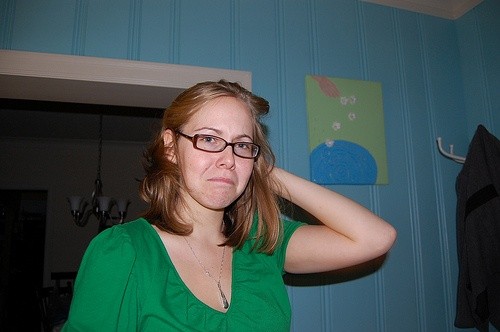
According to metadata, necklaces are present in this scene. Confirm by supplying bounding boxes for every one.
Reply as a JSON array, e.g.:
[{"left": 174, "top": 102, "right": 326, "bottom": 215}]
[{"left": 184, "top": 235, "right": 229, "bottom": 309}]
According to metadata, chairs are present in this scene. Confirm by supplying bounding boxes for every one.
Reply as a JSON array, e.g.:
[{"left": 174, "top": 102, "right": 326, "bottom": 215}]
[{"left": 44, "top": 272, "right": 78, "bottom": 331}]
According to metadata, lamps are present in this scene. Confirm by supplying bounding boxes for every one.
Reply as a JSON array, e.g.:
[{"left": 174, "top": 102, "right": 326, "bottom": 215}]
[{"left": 65, "top": 103, "right": 129, "bottom": 233}]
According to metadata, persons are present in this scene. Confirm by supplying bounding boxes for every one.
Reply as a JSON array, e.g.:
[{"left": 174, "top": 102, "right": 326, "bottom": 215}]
[{"left": 60, "top": 79, "right": 396, "bottom": 332}]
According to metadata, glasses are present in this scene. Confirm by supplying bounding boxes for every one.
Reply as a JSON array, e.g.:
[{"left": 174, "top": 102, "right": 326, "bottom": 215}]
[{"left": 175, "top": 130, "right": 262, "bottom": 159}]
